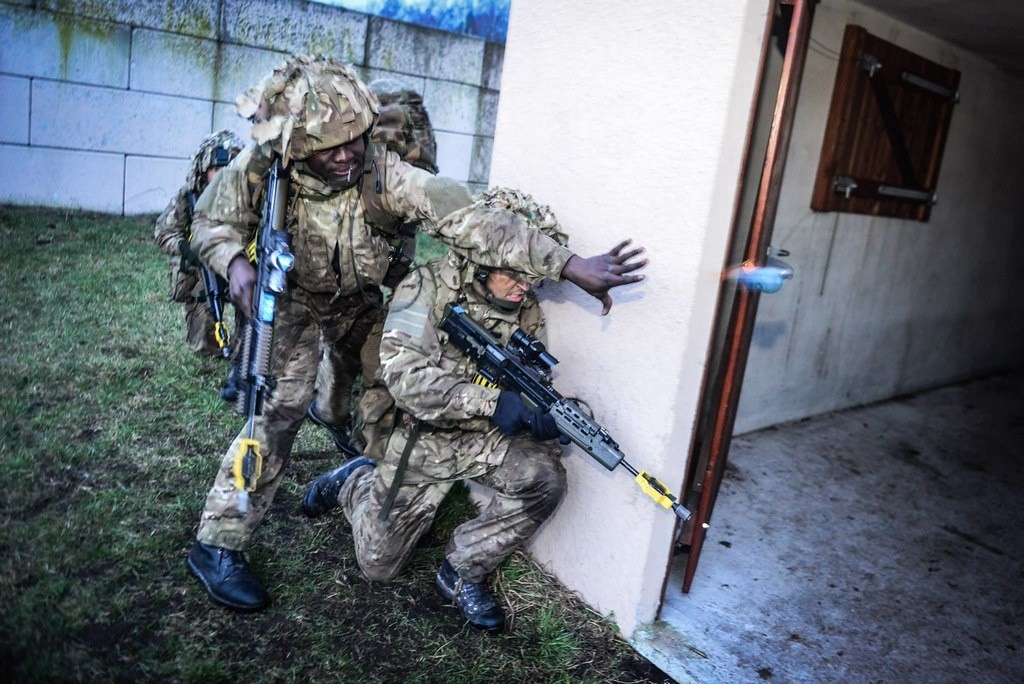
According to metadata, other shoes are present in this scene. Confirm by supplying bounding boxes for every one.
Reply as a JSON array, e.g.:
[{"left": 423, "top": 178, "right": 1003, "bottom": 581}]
[
  {"left": 436, "top": 559, "right": 510, "bottom": 635},
  {"left": 220, "top": 364, "right": 240, "bottom": 401},
  {"left": 303, "top": 457, "right": 376, "bottom": 518}
]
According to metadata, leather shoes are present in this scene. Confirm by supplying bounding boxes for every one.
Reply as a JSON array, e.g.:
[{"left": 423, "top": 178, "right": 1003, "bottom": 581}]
[
  {"left": 308, "top": 399, "right": 366, "bottom": 458},
  {"left": 187, "top": 540, "right": 271, "bottom": 612}
]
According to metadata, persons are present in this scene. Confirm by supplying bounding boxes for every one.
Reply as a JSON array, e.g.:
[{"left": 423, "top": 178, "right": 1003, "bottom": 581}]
[
  {"left": 302, "top": 189, "right": 588, "bottom": 628},
  {"left": 153, "top": 56, "right": 648, "bottom": 632}
]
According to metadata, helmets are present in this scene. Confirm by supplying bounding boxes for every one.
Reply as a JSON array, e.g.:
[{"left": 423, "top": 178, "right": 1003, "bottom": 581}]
[
  {"left": 369, "top": 78, "right": 422, "bottom": 104},
  {"left": 269, "top": 75, "right": 374, "bottom": 160},
  {"left": 468, "top": 193, "right": 562, "bottom": 299},
  {"left": 201, "top": 147, "right": 241, "bottom": 167}
]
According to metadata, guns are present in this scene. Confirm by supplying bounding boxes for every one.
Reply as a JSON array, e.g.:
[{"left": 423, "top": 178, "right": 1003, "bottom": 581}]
[
  {"left": 186, "top": 190, "right": 231, "bottom": 360},
  {"left": 230, "top": 160, "right": 291, "bottom": 495},
  {"left": 433, "top": 301, "right": 693, "bottom": 527}
]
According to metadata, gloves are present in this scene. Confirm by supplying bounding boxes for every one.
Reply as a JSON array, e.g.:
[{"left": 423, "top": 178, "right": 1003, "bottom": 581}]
[{"left": 179, "top": 242, "right": 204, "bottom": 276}]
